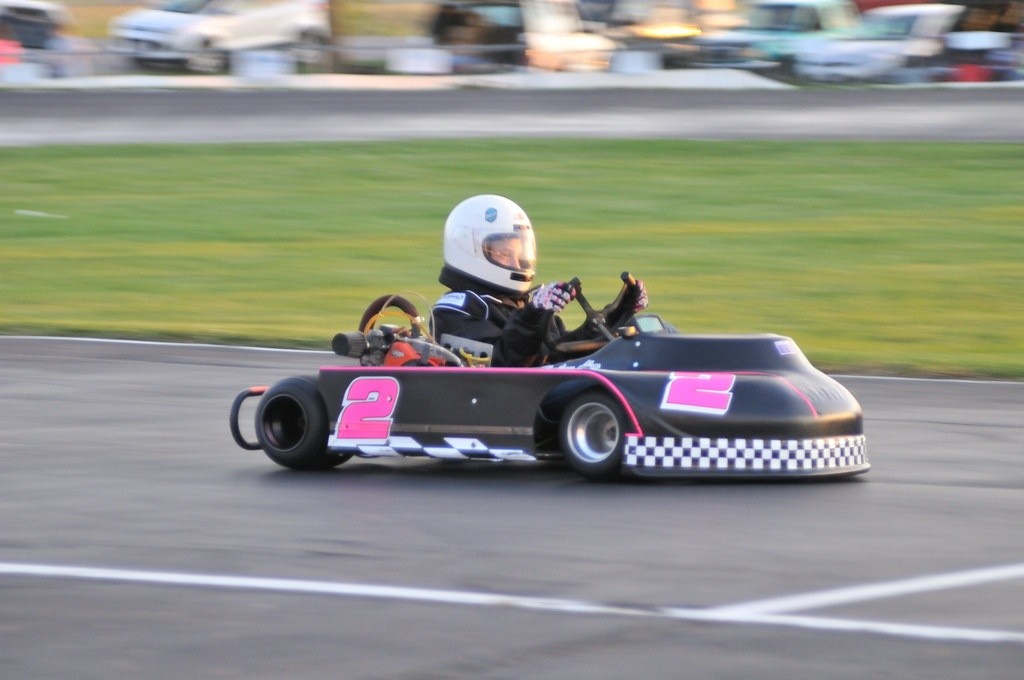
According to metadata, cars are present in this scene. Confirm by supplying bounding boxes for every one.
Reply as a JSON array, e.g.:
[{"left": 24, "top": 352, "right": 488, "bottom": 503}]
[
  {"left": 0, "top": 3, "right": 97, "bottom": 89},
  {"left": 433, "top": 0, "right": 1024, "bottom": 85},
  {"left": 103, "top": 0, "right": 333, "bottom": 76}
]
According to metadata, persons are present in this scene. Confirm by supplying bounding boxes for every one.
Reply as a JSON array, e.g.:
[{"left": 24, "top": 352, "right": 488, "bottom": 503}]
[
  {"left": 0, "top": 20, "right": 72, "bottom": 79},
  {"left": 432, "top": 0, "right": 485, "bottom": 70},
  {"left": 429, "top": 194, "right": 649, "bottom": 369}
]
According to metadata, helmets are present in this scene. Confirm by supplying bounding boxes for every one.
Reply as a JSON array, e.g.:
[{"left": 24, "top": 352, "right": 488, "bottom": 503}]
[{"left": 438, "top": 194, "right": 536, "bottom": 297}]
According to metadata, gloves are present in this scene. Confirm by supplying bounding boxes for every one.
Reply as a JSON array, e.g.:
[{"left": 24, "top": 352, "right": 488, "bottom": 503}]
[
  {"left": 527, "top": 281, "right": 576, "bottom": 313},
  {"left": 612, "top": 280, "right": 648, "bottom": 318}
]
